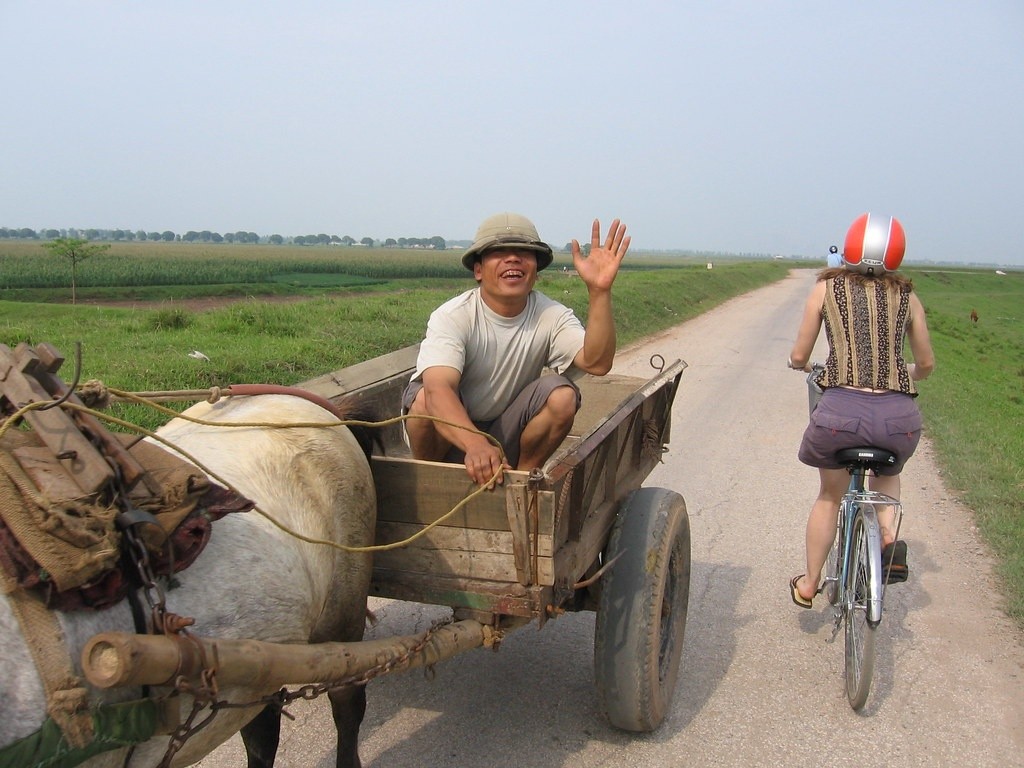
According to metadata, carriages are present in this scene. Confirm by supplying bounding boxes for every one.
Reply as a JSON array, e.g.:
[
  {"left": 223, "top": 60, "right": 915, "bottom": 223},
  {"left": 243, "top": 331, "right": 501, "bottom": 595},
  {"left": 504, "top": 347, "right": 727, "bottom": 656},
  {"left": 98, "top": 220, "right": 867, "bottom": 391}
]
[{"left": 0, "top": 334, "right": 693, "bottom": 768}]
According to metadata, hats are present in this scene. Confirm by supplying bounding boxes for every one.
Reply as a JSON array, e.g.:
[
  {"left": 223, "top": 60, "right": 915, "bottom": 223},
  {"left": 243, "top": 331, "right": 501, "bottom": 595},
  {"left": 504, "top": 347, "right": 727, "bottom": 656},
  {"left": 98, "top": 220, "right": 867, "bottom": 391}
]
[
  {"left": 829, "top": 246, "right": 837, "bottom": 253},
  {"left": 462, "top": 213, "right": 553, "bottom": 273}
]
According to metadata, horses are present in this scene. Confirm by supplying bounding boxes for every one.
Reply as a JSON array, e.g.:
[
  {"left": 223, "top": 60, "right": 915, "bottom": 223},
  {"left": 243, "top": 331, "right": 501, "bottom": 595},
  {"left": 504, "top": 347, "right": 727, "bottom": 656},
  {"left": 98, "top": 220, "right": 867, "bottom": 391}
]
[{"left": 2, "top": 389, "right": 377, "bottom": 767}]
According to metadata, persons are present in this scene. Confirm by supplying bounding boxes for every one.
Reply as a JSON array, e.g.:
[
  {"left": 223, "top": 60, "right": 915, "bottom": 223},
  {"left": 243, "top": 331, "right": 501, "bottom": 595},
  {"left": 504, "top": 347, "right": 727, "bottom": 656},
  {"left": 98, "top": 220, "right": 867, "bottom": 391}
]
[
  {"left": 789, "top": 212, "right": 935, "bottom": 609},
  {"left": 400, "top": 212, "right": 631, "bottom": 492}
]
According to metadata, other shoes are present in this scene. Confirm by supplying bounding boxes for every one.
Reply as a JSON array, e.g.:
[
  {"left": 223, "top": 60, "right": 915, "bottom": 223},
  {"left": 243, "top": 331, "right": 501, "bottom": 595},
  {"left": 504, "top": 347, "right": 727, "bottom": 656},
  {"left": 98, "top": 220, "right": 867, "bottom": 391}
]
[{"left": 881, "top": 540, "right": 908, "bottom": 584}]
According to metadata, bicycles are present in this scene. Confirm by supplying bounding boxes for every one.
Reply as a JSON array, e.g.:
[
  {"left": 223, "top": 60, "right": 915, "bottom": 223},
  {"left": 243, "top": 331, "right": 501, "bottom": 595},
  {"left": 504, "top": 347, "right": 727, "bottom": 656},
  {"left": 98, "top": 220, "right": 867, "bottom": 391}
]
[{"left": 792, "top": 361, "right": 903, "bottom": 711}]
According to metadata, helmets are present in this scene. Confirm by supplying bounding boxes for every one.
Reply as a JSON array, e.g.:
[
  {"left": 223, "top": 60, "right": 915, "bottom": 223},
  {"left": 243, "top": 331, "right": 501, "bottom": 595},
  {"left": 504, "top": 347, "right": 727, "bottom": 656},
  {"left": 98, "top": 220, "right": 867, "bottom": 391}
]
[{"left": 840, "top": 212, "right": 906, "bottom": 276}]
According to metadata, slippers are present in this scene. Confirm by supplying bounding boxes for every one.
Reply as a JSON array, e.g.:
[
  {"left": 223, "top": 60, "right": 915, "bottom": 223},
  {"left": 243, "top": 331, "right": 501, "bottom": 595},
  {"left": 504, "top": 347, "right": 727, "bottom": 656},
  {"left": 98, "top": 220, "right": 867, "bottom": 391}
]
[{"left": 789, "top": 574, "right": 812, "bottom": 609}]
